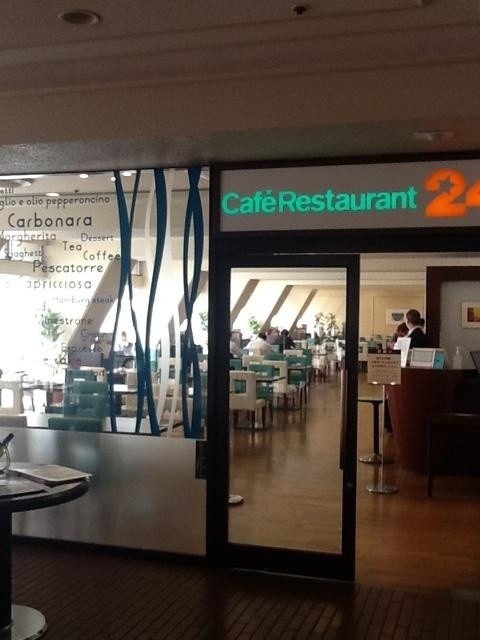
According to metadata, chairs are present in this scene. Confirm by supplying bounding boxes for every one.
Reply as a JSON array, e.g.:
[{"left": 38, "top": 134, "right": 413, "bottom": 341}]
[{"left": 0, "top": 333, "right": 393, "bottom": 432}]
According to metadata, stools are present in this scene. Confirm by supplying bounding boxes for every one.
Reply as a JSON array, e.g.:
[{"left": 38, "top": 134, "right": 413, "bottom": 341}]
[{"left": 357, "top": 399, "right": 394, "bottom": 464}]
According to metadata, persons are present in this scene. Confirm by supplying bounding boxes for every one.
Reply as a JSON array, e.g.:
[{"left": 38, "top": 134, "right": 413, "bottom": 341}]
[
  {"left": 244, "top": 330, "right": 274, "bottom": 356},
  {"left": 271, "top": 328, "right": 296, "bottom": 352},
  {"left": 230, "top": 331, "right": 243, "bottom": 359},
  {"left": 118, "top": 329, "right": 133, "bottom": 368},
  {"left": 392, "top": 322, "right": 409, "bottom": 343},
  {"left": 417, "top": 317, "right": 425, "bottom": 331},
  {"left": 385, "top": 308, "right": 425, "bottom": 355},
  {"left": 265, "top": 328, "right": 278, "bottom": 345}
]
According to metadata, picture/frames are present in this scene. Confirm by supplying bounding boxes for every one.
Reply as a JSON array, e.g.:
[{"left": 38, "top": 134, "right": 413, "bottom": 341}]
[
  {"left": 386, "top": 309, "right": 409, "bottom": 326},
  {"left": 461, "top": 302, "right": 480, "bottom": 328}
]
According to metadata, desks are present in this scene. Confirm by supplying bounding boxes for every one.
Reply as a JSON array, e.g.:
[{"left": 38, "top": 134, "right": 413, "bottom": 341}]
[{"left": 0, "top": 461, "right": 88, "bottom": 640}]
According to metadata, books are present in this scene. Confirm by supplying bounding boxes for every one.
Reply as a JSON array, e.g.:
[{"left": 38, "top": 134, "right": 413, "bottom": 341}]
[
  {"left": 5, "top": 462, "right": 95, "bottom": 486},
  {"left": 0, "top": 480, "right": 45, "bottom": 499}
]
